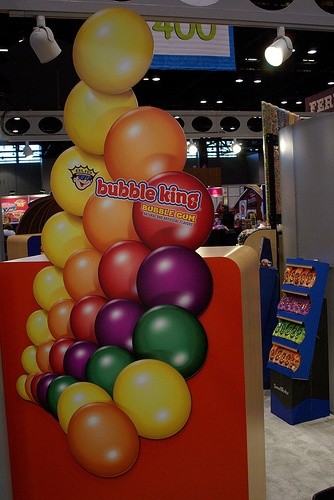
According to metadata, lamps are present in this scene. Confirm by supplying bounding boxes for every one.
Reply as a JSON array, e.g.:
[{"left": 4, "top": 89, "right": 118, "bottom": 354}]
[
  {"left": 188, "top": 139, "right": 197, "bottom": 155},
  {"left": 265, "top": 26, "right": 293, "bottom": 66},
  {"left": 23, "top": 141, "right": 33, "bottom": 157},
  {"left": 30, "top": 16, "right": 61, "bottom": 64},
  {"left": 232, "top": 138, "right": 241, "bottom": 154}
]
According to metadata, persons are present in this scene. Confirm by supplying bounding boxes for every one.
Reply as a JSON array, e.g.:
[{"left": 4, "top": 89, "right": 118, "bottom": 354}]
[
  {"left": 220, "top": 205, "right": 235, "bottom": 230},
  {"left": 3, "top": 223, "right": 15, "bottom": 236}
]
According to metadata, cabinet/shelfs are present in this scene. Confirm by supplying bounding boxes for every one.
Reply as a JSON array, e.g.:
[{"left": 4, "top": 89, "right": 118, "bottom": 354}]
[{"left": 267, "top": 257, "right": 329, "bottom": 381}]
